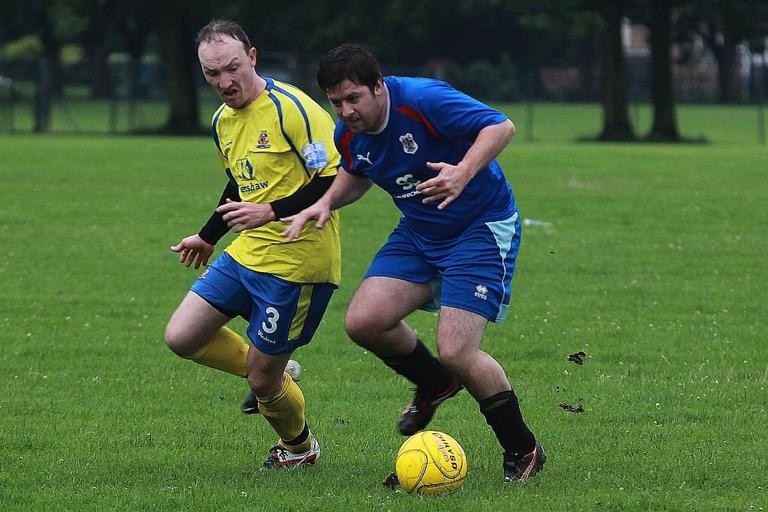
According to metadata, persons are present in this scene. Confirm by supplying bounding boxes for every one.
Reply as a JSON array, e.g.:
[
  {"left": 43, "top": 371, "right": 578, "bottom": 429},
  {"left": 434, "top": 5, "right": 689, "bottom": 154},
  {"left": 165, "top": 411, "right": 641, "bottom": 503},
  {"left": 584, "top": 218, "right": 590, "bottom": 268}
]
[
  {"left": 165, "top": 19, "right": 341, "bottom": 467},
  {"left": 279, "top": 45, "right": 545, "bottom": 483}
]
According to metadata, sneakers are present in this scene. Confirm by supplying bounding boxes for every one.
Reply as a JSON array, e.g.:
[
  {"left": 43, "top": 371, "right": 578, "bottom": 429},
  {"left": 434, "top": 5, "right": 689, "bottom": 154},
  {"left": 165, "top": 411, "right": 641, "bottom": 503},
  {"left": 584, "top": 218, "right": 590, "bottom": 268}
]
[
  {"left": 502, "top": 441, "right": 546, "bottom": 483},
  {"left": 399, "top": 372, "right": 463, "bottom": 436},
  {"left": 240, "top": 359, "right": 302, "bottom": 414},
  {"left": 262, "top": 440, "right": 320, "bottom": 469}
]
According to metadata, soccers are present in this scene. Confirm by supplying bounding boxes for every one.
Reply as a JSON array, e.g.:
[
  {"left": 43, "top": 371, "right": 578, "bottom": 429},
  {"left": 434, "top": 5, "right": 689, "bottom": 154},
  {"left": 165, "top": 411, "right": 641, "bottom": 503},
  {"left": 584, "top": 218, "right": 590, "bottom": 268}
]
[{"left": 396, "top": 431, "right": 467, "bottom": 498}]
